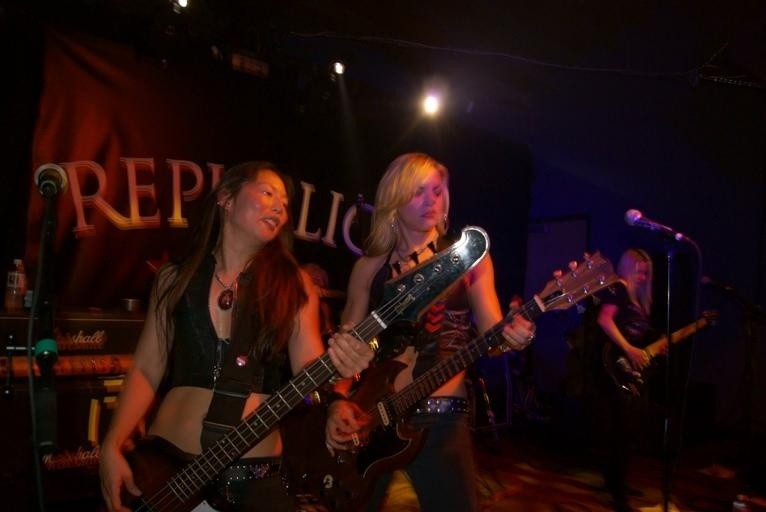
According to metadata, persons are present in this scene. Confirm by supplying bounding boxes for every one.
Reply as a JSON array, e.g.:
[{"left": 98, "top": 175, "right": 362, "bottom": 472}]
[
  {"left": 99, "top": 161, "right": 375, "bottom": 512},
  {"left": 597, "top": 249, "right": 669, "bottom": 512},
  {"left": 325, "top": 152, "right": 537, "bottom": 511}
]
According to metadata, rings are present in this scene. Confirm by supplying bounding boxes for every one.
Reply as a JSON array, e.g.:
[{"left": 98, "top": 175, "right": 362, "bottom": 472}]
[{"left": 528, "top": 336, "right": 535, "bottom": 342}]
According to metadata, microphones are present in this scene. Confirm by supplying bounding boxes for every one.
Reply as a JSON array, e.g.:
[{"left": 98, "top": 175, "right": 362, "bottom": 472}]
[
  {"left": 33, "top": 163, "right": 69, "bottom": 201},
  {"left": 624, "top": 209, "right": 684, "bottom": 243},
  {"left": 32, "top": 336, "right": 59, "bottom": 455},
  {"left": 701, "top": 273, "right": 738, "bottom": 297}
]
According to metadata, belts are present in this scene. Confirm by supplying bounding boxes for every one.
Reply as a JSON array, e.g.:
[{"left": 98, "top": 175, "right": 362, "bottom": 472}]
[
  {"left": 412, "top": 397, "right": 471, "bottom": 416},
  {"left": 215, "top": 460, "right": 281, "bottom": 482}
]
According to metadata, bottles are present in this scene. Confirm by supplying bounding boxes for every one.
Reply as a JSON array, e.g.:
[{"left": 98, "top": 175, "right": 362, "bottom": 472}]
[{"left": 4, "top": 259, "right": 27, "bottom": 315}]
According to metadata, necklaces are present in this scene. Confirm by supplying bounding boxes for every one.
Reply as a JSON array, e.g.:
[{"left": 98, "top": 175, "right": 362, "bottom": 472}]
[{"left": 215, "top": 271, "right": 235, "bottom": 311}]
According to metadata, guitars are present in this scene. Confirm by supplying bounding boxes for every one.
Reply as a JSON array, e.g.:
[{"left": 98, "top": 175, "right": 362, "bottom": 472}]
[
  {"left": 602, "top": 310, "right": 720, "bottom": 398},
  {"left": 284, "top": 249, "right": 618, "bottom": 511},
  {"left": 77, "top": 225, "right": 491, "bottom": 512}
]
